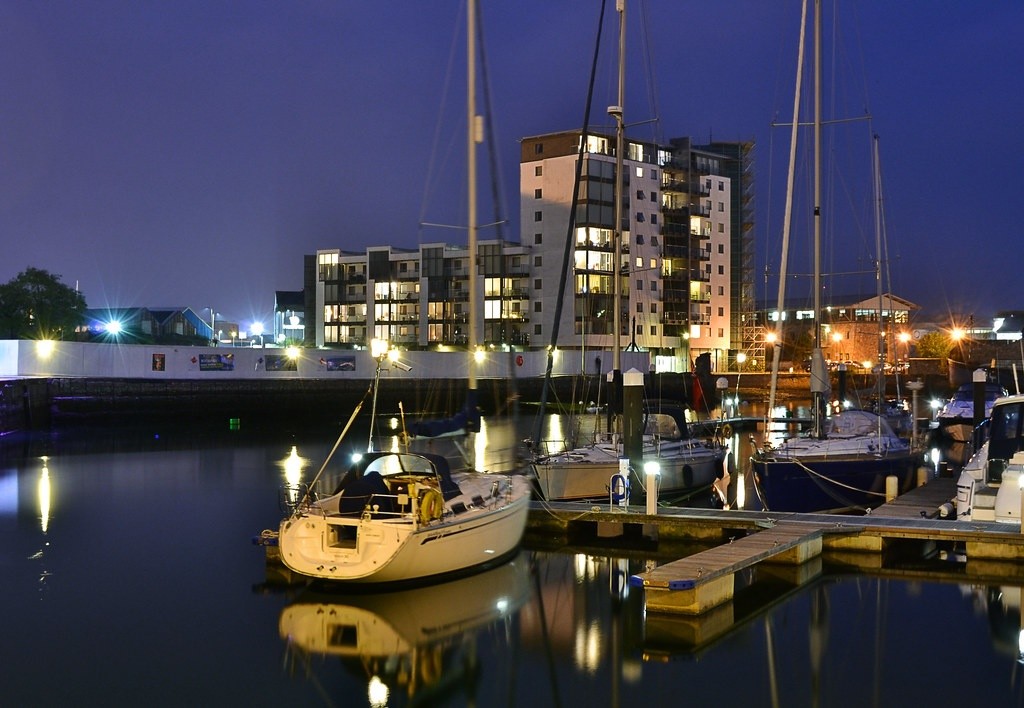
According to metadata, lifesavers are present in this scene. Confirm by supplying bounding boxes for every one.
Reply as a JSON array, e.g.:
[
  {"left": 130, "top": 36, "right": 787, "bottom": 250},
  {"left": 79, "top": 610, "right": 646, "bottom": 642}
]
[
  {"left": 421, "top": 491, "right": 442, "bottom": 522},
  {"left": 609, "top": 474, "right": 627, "bottom": 501}
]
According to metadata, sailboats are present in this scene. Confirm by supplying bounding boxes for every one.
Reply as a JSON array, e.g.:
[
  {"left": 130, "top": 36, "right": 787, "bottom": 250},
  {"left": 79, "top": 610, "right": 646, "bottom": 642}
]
[{"left": 379, "top": 0, "right": 921, "bottom": 514}]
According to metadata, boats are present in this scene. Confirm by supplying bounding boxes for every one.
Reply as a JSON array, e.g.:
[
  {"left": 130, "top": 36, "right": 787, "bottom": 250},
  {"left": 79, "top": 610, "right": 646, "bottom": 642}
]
[
  {"left": 280, "top": 546, "right": 539, "bottom": 708},
  {"left": 936, "top": 382, "right": 1009, "bottom": 442},
  {"left": 955, "top": 393, "right": 1024, "bottom": 524},
  {"left": 277, "top": 401, "right": 533, "bottom": 585}
]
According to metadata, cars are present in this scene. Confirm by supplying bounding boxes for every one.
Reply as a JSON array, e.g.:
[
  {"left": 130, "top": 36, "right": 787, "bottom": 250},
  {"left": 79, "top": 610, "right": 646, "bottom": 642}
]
[
  {"left": 826, "top": 363, "right": 839, "bottom": 371},
  {"left": 845, "top": 360, "right": 864, "bottom": 369},
  {"left": 872, "top": 362, "right": 902, "bottom": 372}
]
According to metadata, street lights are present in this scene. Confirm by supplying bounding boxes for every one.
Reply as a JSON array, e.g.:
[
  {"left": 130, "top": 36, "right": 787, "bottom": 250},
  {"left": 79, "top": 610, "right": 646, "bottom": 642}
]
[{"left": 951, "top": 328, "right": 965, "bottom": 359}]
